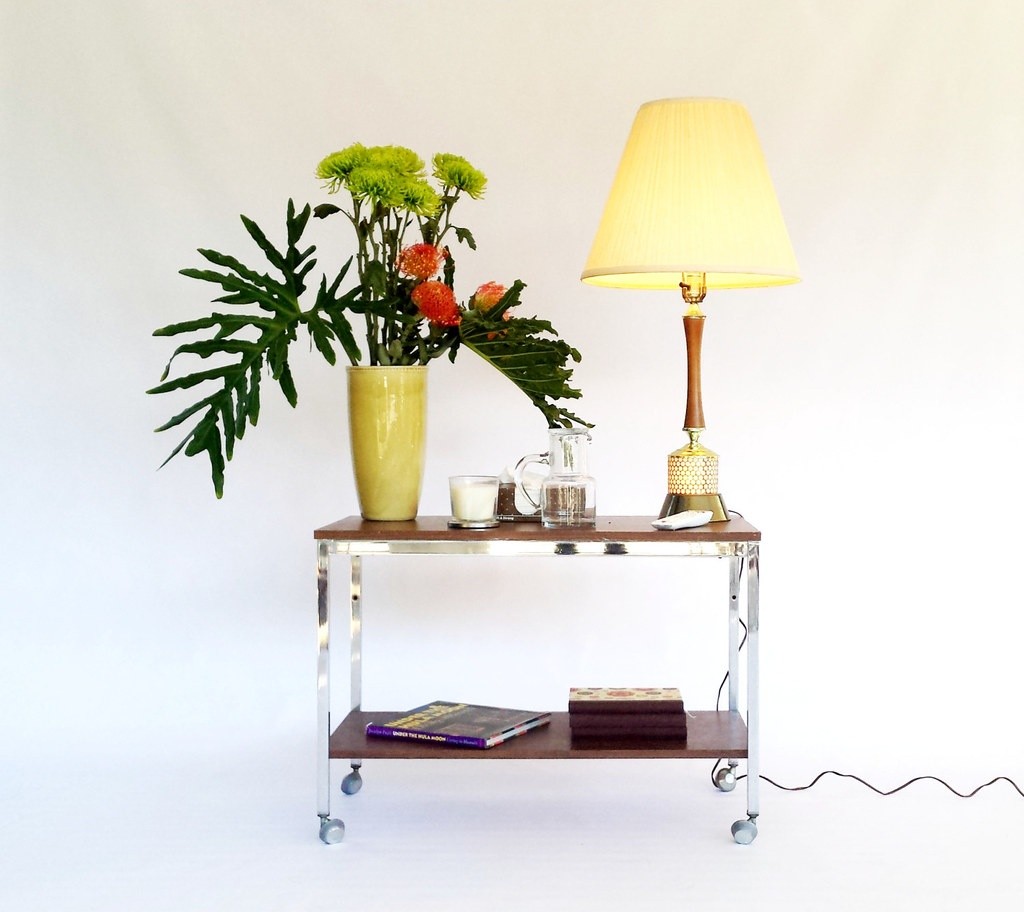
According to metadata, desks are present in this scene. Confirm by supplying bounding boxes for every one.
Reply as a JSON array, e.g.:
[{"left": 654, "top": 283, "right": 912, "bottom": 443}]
[{"left": 313, "top": 514, "right": 761, "bottom": 846}]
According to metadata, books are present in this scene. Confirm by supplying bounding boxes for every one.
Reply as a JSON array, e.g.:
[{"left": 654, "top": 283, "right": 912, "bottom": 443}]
[
  {"left": 367, "top": 701, "right": 552, "bottom": 749},
  {"left": 569, "top": 688, "right": 687, "bottom": 738}
]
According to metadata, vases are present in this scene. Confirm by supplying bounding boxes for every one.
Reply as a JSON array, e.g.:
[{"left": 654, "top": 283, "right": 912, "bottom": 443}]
[{"left": 347, "top": 365, "right": 429, "bottom": 521}]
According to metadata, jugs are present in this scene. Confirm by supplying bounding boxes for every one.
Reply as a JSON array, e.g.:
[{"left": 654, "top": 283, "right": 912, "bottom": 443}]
[{"left": 514, "top": 428, "right": 597, "bottom": 528}]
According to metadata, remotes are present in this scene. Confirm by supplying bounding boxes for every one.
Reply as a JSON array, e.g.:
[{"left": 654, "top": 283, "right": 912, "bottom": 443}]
[{"left": 649, "top": 510, "right": 713, "bottom": 530}]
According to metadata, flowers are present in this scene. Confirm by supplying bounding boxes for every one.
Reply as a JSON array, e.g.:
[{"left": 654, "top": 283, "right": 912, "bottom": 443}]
[{"left": 145, "top": 142, "right": 595, "bottom": 498}]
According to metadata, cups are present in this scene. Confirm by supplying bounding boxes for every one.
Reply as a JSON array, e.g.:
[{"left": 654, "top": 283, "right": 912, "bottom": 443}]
[{"left": 449, "top": 475, "right": 499, "bottom": 523}]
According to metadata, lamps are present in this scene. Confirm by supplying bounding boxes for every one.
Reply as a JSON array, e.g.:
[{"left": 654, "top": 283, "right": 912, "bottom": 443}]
[{"left": 580, "top": 98, "right": 801, "bottom": 522}]
